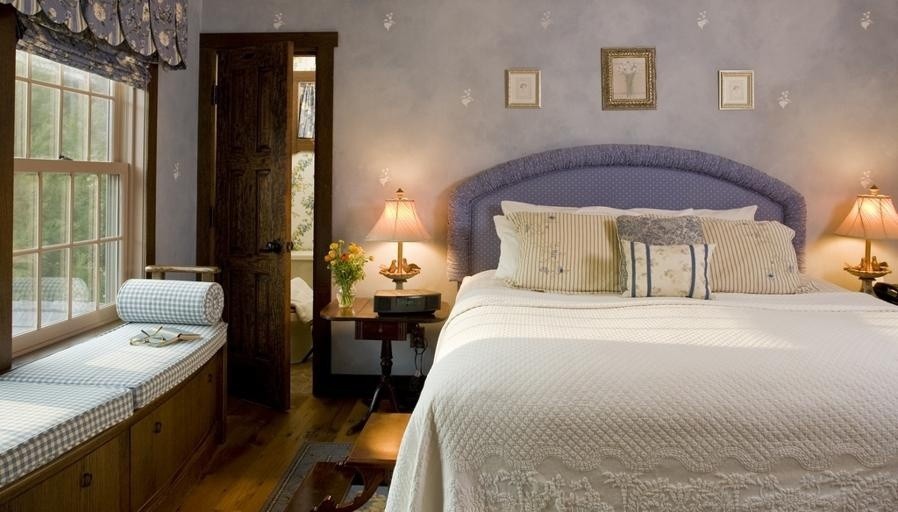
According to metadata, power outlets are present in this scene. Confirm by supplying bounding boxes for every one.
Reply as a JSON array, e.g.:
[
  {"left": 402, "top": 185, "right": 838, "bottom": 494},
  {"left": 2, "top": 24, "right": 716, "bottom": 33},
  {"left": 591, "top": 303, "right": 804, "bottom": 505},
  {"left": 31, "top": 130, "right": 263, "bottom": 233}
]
[{"left": 410, "top": 327, "right": 425, "bottom": 349}]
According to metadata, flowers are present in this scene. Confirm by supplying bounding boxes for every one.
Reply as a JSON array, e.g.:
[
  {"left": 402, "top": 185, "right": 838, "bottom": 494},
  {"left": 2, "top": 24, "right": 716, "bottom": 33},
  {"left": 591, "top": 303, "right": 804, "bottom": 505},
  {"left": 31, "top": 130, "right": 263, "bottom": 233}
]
[{"left": 324, "top": 240, "right": 374, "bottom": 305}]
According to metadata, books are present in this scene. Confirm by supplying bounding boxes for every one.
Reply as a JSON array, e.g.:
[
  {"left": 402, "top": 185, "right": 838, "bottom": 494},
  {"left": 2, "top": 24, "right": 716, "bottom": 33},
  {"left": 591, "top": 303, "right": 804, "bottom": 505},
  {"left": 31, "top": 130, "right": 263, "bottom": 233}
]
[{"left": 128, "top": 327, "right": 202, "bottom": 347}]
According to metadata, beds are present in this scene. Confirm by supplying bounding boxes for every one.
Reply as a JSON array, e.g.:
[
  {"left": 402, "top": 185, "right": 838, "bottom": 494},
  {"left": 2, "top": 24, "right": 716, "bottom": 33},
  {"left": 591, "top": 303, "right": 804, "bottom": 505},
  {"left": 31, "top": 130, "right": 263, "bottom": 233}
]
[{"left": 382, "top": 144, "right": 898, "bottom": 512}]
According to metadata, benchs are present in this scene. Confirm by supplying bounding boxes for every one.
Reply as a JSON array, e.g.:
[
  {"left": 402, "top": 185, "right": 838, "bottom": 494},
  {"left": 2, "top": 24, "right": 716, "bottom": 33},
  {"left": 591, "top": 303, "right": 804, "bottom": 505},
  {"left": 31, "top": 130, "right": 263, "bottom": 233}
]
[{"left": 0, "top": 265, "right": 230, "bottom": 512}]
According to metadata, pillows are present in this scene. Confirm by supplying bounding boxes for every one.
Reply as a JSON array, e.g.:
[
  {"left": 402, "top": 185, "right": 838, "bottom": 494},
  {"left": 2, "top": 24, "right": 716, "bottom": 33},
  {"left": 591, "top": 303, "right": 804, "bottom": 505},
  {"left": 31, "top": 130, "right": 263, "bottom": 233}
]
[
  {"left": 114, "top": 278, "right": 225, "bottom": 326},
  {"left": 504, "top": 209, "right": 818, "bottom": 294},
  {"left": 492, "top": 213, "right": 524, "bottom": 290},
  {"left": 500, "top": 200, "right": 759, "bottom": 227},
  {"left": 614, "top": 213, "right": 709, "bottom": 292},
  {"left": 620, "top": 238, "right": 720, "bottom": 301}
]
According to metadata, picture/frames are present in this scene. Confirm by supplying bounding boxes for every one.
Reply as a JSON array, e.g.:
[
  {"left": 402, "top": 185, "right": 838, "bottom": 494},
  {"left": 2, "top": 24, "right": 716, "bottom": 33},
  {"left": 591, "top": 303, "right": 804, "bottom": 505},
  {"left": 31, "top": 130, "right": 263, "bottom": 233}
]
[
  {"left": 601, "top": 48, "right": 656, "bottom": 109},
  {"left": 505, "top": 69, "right": 542, "bottom": 108},
  {"left": 719, "top": 70, "right": 754, "bottom": 110}
]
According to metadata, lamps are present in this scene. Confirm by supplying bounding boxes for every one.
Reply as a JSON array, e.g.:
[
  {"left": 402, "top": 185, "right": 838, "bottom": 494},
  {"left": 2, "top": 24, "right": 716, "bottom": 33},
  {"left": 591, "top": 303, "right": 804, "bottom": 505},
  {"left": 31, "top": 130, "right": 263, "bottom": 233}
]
[
  {"left": 833, "top": 184, "right": 898, "bottom": 297},
  {"left": 363, "top": 188, "right": 432, "bottom": 289}
]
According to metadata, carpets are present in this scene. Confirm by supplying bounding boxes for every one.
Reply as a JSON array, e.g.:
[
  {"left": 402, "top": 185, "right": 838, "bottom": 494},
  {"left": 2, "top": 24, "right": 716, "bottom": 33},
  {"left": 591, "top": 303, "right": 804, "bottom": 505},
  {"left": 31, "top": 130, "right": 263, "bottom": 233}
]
[{"left": 259, "top": 440, "right": 389, "bottom": 512}]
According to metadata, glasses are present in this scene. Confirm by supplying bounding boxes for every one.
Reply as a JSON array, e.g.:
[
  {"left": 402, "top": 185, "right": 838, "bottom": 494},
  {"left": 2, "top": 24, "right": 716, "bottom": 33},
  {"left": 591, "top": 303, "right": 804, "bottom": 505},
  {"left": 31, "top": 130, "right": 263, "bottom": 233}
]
[{"left": 130, "top": 326, "right": 165, "bottom": 345}]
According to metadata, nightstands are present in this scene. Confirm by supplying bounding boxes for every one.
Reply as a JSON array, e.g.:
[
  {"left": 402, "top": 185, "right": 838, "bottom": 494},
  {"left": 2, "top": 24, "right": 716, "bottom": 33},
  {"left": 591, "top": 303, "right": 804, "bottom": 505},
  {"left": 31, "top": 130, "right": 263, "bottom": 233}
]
[{"left": 320, "top": 297, "right": 451, "bottom": 436}]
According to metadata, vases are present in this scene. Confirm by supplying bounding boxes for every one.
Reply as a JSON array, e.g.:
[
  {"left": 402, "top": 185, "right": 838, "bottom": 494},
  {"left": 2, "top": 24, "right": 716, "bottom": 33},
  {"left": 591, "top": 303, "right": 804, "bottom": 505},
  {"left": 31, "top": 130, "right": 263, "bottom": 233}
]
[{"left": 335, "top": 285, "right": 356, "bottom": 309}]
